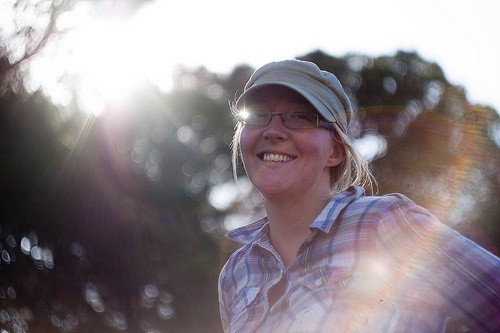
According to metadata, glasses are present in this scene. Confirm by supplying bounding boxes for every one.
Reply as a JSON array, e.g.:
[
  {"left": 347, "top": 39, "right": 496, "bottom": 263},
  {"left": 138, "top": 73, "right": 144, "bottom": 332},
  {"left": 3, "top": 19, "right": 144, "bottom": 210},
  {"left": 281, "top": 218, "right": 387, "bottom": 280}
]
[{"left": 237, "top": 106, "right": 338, "bottom": 132}]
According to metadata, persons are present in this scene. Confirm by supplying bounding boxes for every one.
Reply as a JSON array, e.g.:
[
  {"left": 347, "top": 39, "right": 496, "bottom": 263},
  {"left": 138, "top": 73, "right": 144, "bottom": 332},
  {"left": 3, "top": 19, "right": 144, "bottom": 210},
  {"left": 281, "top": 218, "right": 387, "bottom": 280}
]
[{"left": 216, "top": 58, "right": 500, "bottom": 333}]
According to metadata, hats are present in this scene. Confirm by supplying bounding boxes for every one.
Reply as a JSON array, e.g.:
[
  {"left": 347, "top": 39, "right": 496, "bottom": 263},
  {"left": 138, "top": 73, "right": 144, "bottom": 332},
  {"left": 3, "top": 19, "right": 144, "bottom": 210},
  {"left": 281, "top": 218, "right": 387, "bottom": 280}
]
[{"left": 234, "top": 58, "right": 353, "bottom": 136}]
